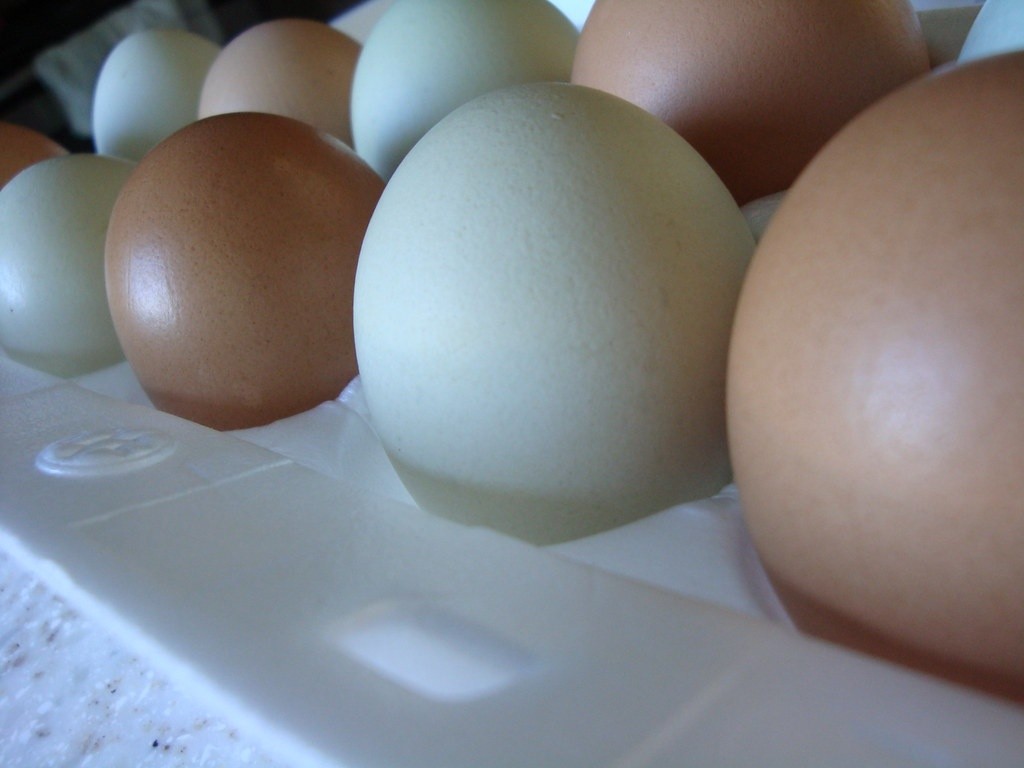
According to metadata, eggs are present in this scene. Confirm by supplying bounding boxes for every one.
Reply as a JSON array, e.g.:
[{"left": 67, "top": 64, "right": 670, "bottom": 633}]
[{"left": 0, "top": 0, "right": 1024, "bottom": 707}]
